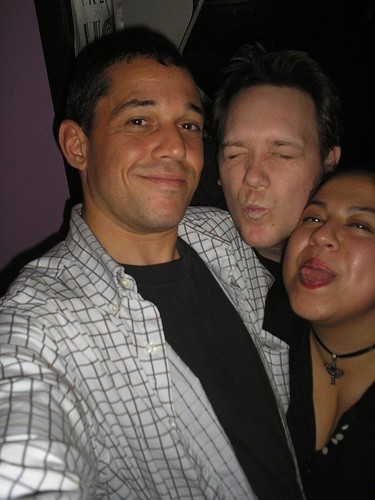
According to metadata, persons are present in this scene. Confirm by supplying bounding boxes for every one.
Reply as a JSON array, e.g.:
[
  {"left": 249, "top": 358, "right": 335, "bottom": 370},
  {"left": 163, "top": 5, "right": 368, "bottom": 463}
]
[
  {"left": 0, "top": 23, "right": 304, "bottom": 500},
  {"left": 212, "top": 42, "right": 342, "bottom": 280},
  {"left": 261, "top": 164, "right": 375, "bottom": 500}
]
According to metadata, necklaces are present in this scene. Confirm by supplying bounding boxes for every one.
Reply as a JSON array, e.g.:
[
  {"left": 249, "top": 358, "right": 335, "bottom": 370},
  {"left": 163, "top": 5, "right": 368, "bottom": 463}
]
[{"left": 310, "top": 324, "right": 375, "bottom": 384}]
